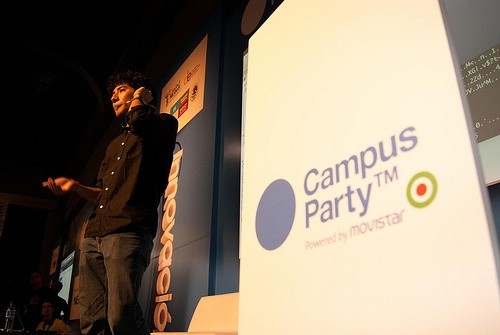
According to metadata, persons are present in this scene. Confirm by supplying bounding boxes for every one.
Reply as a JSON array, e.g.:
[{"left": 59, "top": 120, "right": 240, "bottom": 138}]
[
  {"left": 37, "top": 65, "right": 178, "bottom": 335},
  {"left": 14, "top": 269, "right": 70, "bottom": 335}
]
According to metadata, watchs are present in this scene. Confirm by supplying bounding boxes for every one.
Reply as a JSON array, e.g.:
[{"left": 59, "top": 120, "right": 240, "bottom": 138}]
[{"left": 131, "top": 94, "right": 147, "bottom": 106}]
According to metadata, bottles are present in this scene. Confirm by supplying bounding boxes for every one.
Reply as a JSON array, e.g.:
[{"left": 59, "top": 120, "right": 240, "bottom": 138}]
[{"left": 3, "top": 300, "right": 16, "bottom": 331}]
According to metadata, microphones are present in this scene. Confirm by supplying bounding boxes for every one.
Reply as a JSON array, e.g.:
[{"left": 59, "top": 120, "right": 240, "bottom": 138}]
[{"left": 126, "top": 98, "right": 134, "bottom": 104}]
